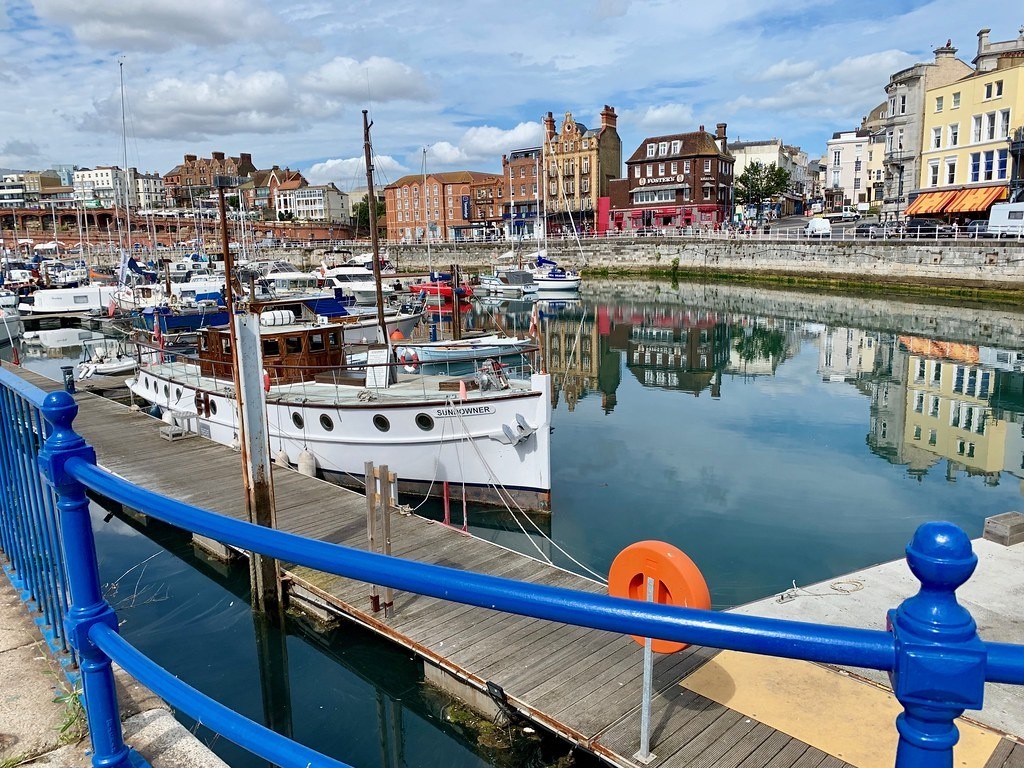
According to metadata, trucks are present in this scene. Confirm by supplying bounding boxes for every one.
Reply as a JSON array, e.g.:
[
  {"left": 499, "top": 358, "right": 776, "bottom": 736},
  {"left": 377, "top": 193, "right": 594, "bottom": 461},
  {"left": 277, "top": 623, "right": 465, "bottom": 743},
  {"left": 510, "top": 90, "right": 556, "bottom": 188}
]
[
  {"left": 803, "top": 218, "right": 831, "bottom": 237},
  {"left": 811, "top": 203, "right": 823, "bottom": 214},
  {"left": 821, "top": 205, "right": 860, "bottom": 224},
  {"left": 987, "top": 203, "right": 1024, "bottom": 238}
]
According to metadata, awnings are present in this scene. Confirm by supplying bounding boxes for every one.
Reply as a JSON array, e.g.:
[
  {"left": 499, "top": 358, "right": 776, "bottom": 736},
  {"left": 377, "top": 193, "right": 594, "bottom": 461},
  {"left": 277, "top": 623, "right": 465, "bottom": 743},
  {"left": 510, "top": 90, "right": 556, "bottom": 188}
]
[
  {"left": 944, "top": 185, "right": 1006, "bottom": 213},
  {"left": 903, "top": 190, "right": 959, "bottom": 213}
]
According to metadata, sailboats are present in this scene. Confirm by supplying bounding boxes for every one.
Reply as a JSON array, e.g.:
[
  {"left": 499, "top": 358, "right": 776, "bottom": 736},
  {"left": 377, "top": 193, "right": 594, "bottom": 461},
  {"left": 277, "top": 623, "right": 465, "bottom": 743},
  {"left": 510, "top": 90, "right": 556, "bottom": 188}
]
[{"left": 0, "top": 54, "right": 590, "bottom": 346}]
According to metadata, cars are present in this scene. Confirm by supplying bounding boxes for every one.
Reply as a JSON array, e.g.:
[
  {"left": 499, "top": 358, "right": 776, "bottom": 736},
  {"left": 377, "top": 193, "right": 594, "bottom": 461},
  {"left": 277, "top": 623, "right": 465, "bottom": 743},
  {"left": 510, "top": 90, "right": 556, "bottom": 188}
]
[{"left": 856, "top": 217, "right": 956, "bottom": 238}]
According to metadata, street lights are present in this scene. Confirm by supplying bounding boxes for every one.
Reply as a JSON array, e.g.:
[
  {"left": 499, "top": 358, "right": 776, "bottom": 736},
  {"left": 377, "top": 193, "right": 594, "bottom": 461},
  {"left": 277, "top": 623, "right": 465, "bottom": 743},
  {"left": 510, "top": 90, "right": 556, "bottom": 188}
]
[
  {"left": 1004, "top": 126, "right": 1024, "bottom": 203},
  {"left": 889, "top": 142, "right": 903, "bottom": 222}
]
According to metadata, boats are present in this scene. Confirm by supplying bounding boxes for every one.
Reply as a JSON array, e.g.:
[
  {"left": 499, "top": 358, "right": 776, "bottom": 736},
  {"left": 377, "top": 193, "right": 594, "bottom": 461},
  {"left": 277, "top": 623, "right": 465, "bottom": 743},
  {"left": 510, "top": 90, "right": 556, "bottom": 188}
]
[
  {"left": 387, "top": 263, "right": 544, "bottom": 364},
  {"left": 122, "top": 109, "right": 556, "bottom": 517},
  {"left": 76, "top": 338, "right": 142, "bottom": 381}
]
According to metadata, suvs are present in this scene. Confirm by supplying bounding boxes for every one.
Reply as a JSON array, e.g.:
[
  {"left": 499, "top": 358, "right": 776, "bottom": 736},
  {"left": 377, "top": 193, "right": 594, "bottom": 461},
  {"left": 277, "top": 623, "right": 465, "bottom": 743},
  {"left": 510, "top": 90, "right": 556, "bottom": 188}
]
[{"left": 967, "top": 219, "right": 1002, "bottom": 238}]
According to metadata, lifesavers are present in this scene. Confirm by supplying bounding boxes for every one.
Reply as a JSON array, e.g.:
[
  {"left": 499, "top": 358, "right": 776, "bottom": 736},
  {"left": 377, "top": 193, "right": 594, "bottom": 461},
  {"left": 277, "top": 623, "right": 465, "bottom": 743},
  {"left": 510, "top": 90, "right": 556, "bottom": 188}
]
[
  {"left": 263, "top": 368, "right": 270, "bottom": 397},
  {"left": 401, "top": 347, "right": 418, "bottom": 372}
]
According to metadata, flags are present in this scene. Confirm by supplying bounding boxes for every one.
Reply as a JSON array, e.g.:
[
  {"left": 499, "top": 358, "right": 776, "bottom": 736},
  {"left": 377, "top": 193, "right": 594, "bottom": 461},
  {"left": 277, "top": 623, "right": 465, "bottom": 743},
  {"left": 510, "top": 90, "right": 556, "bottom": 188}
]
[{"left": 108, "top": 295, "right": 117, "bottom": 317}]
